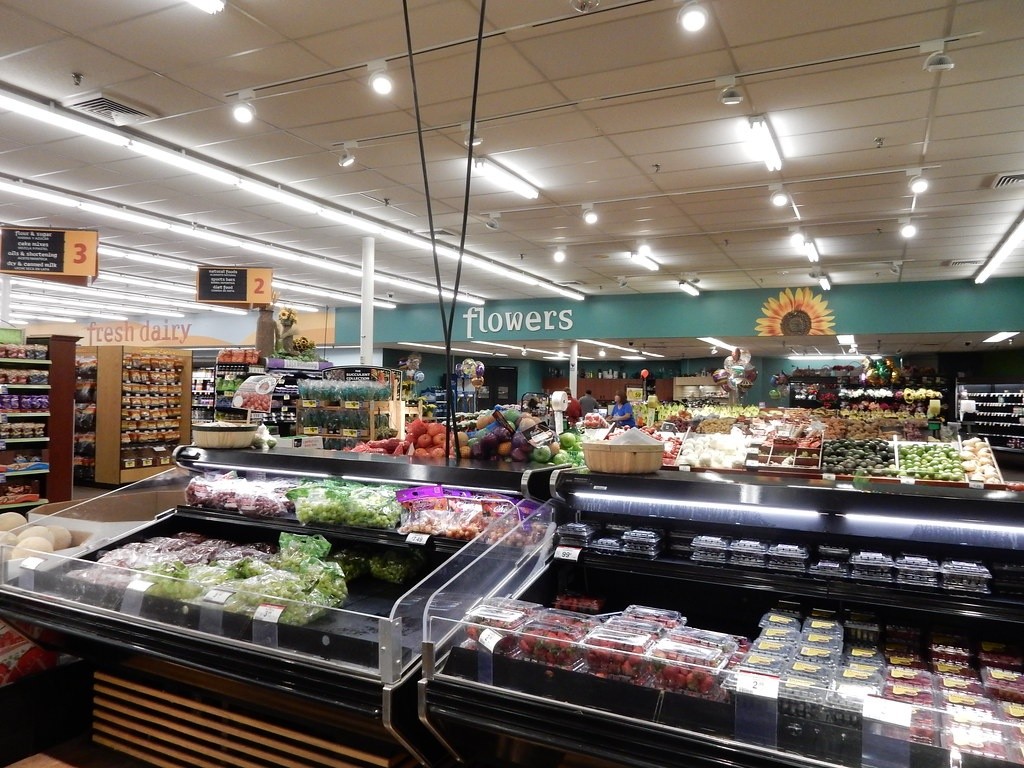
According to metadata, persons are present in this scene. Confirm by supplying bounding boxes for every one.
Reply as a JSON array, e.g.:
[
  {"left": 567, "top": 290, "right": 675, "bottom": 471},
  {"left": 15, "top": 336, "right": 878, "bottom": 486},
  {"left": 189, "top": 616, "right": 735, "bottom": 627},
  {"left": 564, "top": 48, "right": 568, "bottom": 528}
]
[
  {"left": 579, "top": 390, "right": 601, "bottom": 421},
  {"left": 816, "top": 380, "right": 841, "bottom": 409},
  {"left": 563, "top": 386, "right": 582, "bottom": 428},
  {"left": 606, "top": 389, "right": 636, "bottom": 428}
]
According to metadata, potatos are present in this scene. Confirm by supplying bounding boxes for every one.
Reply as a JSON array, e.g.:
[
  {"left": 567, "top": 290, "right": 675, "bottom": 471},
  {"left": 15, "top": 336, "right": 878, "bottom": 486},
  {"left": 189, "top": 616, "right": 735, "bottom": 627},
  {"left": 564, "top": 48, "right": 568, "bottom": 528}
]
[{"left": 825, "top": 416, "right": 882, "bottom": 440}]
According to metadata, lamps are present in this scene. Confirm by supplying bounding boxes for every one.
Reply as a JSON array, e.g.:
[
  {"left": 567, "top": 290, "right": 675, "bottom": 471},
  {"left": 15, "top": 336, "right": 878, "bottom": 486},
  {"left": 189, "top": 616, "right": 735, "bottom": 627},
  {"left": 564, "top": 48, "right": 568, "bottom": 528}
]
[
  {"left": 889, "top": 261, "right": 903, "bottom": 275},
  {"left": 819, "top": 275, "right": 831, "bottom": 290},
  {"left": 767, "top": 184, "right": 787, "bottom": 206},
  {"left": 679, "top": 281, "right": 701, "bottom": 297},
  {"left": 712, "top": 77, "right": 744, "bottom": 104},
  {"left": 788, "top": 226, "right": 804, "bottom": 246},
  {"left": 898, "top": 218, "right": 916, "bottom": 238},
  {"left": 748, "top": 114, "right": 783, "bottom": 171},
  {"left": 906, "top": 169, "right": 929, "bottom": 193},
  {"left": 631, "top": 252, "right": 662, "bottom": 272},
  {"left": 0, "top": 0, "right": 600, "bottom": 333},
  {"left": 676, "top": 0, "right": 709, "bottom": 32},
  {"left": 804, "top": 240, "right": 820, "bottom": 263},
  {"left": 919, "top": 42, "right": 955, "bottom": 74}
]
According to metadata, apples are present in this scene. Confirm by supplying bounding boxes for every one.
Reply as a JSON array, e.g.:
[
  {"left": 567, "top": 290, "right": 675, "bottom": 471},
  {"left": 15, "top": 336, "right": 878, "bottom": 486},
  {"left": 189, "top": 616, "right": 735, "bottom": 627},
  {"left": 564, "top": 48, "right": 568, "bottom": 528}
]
[
  {"left": 585, "top": 412, "right": 684, "bottom": 466},
  {"left": 394, "top": 408, "right": 586, "bottom": 464}
]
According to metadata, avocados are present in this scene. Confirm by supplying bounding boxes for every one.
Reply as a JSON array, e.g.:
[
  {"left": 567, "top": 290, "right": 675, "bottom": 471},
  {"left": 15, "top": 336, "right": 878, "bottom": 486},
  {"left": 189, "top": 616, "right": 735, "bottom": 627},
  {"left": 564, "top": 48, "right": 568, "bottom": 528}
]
[{"left": 821, "top": 437, "right": 897, "bottom": 477}]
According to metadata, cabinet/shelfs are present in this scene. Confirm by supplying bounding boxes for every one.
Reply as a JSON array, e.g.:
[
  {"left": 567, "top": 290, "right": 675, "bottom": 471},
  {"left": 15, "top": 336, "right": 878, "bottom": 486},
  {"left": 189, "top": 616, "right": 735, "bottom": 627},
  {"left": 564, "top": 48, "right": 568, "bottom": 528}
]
[{"left": 0, "top": 332, "right": 1024, "bottom": 768}]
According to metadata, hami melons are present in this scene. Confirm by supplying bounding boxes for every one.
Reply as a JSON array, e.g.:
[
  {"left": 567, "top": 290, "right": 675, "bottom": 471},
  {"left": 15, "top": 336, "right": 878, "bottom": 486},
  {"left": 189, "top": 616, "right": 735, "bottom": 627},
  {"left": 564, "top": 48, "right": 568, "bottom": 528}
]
[{"left": 0, "top": 512, "right": 73, "bottom": 561}]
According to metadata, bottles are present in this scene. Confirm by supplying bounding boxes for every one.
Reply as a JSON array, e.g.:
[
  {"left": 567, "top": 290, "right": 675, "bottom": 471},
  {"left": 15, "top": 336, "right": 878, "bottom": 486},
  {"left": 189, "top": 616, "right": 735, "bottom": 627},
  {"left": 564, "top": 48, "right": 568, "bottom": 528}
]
[{"left": 217, "top": 366, "right": 244, "bottom": 375}]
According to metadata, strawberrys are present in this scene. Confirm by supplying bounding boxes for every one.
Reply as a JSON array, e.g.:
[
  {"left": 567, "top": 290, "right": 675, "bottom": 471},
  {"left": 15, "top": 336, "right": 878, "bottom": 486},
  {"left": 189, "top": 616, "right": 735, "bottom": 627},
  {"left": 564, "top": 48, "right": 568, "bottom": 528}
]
[{"left": 462, "top": 588, "right": 753, "bottom": 705}]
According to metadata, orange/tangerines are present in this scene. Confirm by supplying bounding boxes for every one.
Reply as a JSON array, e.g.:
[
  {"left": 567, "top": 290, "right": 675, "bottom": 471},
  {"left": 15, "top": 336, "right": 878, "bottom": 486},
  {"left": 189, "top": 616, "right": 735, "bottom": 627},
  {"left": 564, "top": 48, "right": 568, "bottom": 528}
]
[{"left": 898, "top": 443, "right": 964, "bottom": 481}]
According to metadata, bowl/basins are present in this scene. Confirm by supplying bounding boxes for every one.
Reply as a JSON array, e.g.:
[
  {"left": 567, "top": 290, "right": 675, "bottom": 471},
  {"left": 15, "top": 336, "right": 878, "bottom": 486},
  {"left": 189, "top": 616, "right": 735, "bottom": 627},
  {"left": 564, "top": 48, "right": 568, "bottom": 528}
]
[
  {"left": 191, "top": 425, "right": 258, "bottom": 449},
  {"left": 583, "top": 443, "right": 664, "bottom": 474}
]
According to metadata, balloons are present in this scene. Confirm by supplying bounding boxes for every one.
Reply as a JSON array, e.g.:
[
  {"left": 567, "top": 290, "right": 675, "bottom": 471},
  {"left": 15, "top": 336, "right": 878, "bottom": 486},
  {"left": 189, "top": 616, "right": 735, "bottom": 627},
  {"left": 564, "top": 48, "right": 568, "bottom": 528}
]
[{"left": 407, "top": 348, "right": 911, "bottom": 400}]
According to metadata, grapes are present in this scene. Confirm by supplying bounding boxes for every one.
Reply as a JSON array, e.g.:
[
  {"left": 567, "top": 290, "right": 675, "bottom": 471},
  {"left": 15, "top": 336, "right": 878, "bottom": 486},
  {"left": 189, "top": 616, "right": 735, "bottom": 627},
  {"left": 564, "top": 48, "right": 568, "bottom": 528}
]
[{"left": 59, "top": 474, "right": 548, "bottom": 627}]
